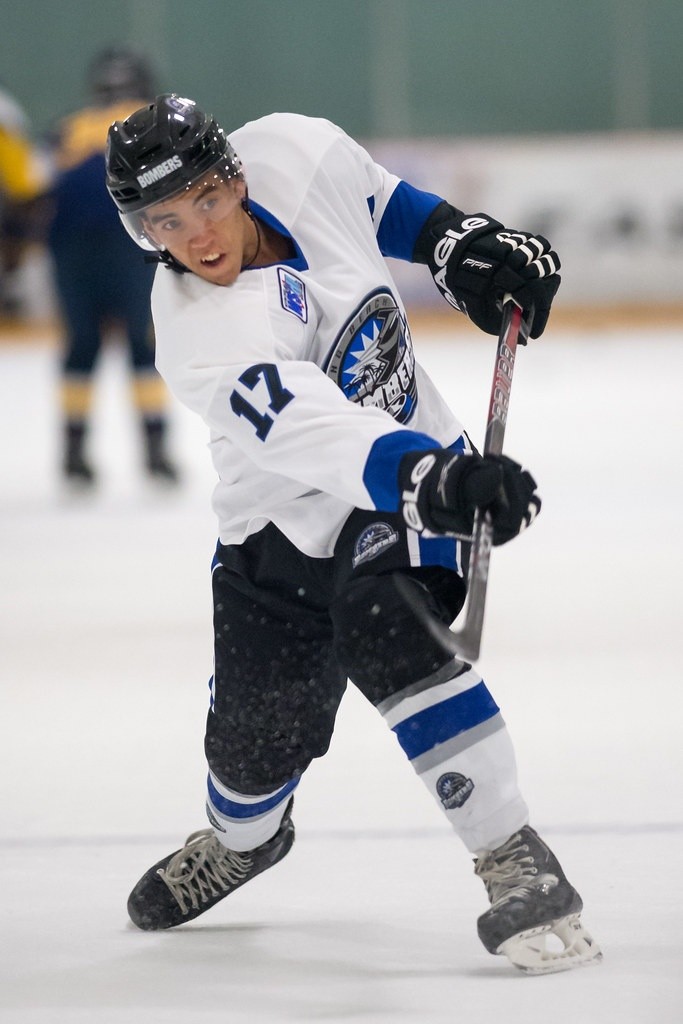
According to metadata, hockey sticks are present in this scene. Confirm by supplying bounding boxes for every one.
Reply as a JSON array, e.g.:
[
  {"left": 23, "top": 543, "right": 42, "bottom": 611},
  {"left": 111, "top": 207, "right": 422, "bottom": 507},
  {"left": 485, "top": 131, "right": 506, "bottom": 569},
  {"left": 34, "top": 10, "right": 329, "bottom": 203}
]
[{"left": 387, "top": 290, "right": 524, "bottom": 664}]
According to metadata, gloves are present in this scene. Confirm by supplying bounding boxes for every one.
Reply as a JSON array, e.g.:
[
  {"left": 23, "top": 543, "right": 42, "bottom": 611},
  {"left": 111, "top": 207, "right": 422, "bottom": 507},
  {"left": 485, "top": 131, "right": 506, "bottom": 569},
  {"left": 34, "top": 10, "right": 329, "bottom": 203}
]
[
  {"left": 398, "top": 450, "right": 541, "bottom": 547},
  {"left": 425, "top": 212, "right": 561, "bottom": 346}
]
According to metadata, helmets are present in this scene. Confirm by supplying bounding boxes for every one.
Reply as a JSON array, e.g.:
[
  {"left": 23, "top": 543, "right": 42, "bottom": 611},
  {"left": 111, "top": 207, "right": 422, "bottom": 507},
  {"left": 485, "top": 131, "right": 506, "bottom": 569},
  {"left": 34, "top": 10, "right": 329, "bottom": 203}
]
[
  {"left": 105, "top": 95, "right": 229, "bottom": 214},
  {"left": 96, "top": 51, "right": 156, "bottom": 91}
]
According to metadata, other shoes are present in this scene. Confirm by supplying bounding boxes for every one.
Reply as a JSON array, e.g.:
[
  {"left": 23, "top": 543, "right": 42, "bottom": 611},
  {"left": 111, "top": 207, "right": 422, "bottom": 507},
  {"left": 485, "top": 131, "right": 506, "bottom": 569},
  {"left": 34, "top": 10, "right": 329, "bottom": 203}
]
[
  {"left": 65, "top": 450, "right": 95, "bottom": 482},
  {"left": 148, "top": 453, "right": 175, "bottom": 481}
]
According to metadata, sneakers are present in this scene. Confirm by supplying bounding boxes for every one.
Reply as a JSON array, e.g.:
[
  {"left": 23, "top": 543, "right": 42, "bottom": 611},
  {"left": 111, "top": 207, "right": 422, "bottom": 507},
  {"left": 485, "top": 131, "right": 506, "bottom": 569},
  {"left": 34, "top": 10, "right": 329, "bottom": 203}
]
[
  {"left": 474, "top": 826, "right": 601, "bottom": 972},
  {"left": 127, "top": 797, "right": 294, "bottom": 930}
]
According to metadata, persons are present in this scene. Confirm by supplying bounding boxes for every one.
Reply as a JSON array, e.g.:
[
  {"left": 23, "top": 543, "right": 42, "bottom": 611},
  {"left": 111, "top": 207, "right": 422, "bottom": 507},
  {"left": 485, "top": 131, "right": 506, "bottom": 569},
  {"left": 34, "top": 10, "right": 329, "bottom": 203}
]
[
  {"left": 0, "top": 46, "right": 181, "bottom": 485},
  {"left": 107, "top": 95, "right": 605, "bottom": 976}
]
